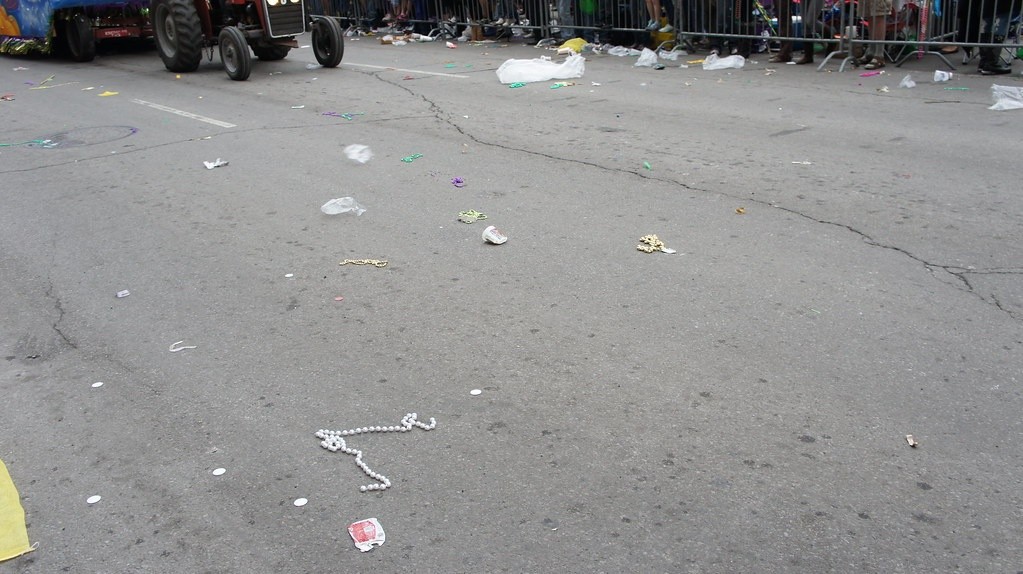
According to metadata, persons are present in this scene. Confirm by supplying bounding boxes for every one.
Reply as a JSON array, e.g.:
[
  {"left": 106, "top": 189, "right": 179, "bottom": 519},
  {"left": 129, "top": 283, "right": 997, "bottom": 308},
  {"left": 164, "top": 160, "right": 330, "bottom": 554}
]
[{"left": 309, "top": 1, "right": 1021, "bottom": 70}]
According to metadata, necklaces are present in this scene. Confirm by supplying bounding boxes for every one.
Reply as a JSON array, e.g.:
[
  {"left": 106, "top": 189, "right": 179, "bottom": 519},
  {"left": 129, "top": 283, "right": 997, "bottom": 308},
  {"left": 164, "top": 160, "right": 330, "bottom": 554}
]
[
  {"left": 340, "top": 257, "right": 388, "bottom": 269},
  {"left": 314, "top": 413, "right": 436, "bottom": 493}
]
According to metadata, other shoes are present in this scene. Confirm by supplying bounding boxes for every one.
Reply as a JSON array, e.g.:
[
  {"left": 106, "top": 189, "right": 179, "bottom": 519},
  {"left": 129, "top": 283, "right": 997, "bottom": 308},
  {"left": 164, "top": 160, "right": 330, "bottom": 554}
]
[{"left": 343, "top": 16, "right": 1011, "bottom": 75}]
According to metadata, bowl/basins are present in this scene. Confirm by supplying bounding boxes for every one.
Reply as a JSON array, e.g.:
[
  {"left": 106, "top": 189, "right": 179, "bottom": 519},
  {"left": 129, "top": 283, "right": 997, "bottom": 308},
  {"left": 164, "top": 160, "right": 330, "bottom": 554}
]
[{"left": 845, "top": 25, "right": 859, "bottom": 39}]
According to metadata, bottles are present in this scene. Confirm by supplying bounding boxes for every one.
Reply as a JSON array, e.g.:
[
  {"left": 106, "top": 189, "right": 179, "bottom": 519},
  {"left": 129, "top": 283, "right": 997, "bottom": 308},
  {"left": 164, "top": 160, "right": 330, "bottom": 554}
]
[{"left": 1016, "top": 26, "right": 1023, "bottom": 58}]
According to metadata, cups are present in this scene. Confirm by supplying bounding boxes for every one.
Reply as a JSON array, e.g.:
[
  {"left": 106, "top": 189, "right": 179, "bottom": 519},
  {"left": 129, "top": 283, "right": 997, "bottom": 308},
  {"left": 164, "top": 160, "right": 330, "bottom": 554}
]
[{"left": 482, "top": 226, "right": 507, "bottom": 244}]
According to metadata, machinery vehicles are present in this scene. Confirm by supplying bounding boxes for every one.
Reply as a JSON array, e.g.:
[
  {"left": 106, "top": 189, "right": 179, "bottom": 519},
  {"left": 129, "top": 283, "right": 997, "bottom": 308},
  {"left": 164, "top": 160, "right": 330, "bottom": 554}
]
[{"left": 48, "top": 0, "right": 345, "bottom": 86}]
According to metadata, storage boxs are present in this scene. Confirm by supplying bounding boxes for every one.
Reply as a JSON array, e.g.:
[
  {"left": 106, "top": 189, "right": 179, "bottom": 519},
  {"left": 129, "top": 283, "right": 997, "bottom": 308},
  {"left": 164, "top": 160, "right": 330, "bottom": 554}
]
[{"left": 764, "top": 16, "right": 804, "bottom": 51}]
